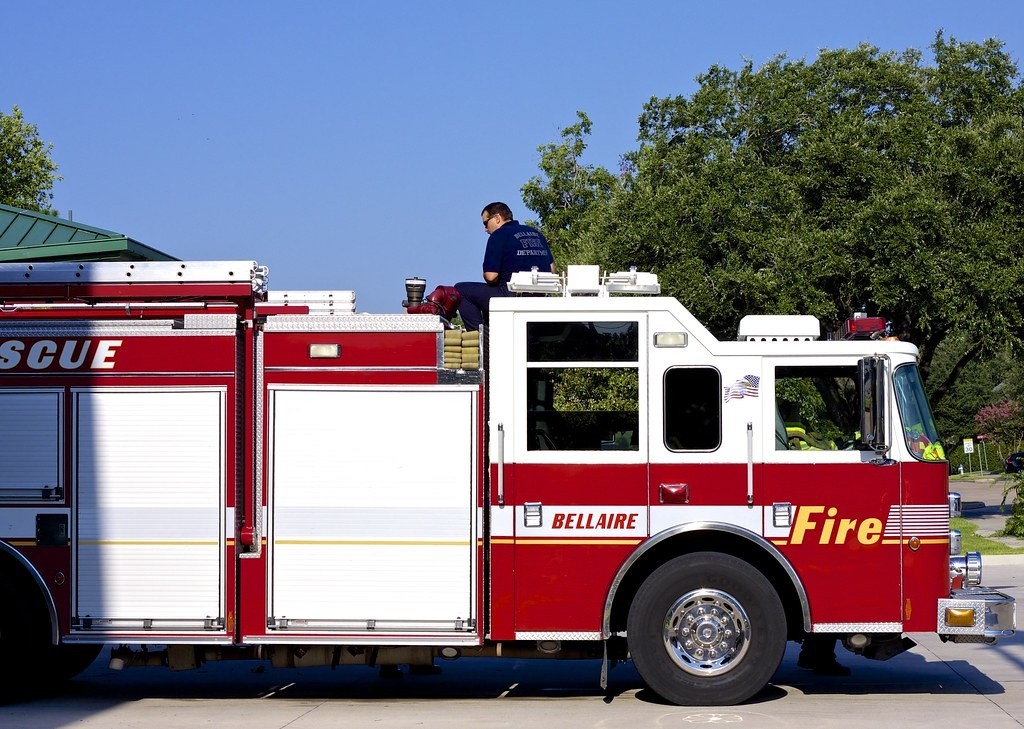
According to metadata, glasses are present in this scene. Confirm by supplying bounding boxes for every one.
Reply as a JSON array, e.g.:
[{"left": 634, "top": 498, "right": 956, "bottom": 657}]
[{"left": 483, "top": 216, "right": 495, "bottom": 227}]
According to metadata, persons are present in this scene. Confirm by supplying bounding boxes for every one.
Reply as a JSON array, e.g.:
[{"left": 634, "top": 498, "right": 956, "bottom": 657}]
[{"left": 454, "top": 202, "right": 553, "bottom": 330}]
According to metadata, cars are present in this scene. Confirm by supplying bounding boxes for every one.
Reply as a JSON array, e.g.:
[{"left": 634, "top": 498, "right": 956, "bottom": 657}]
[{"left": 1004, "top": 453, "right": 1024, "bottom": 474}]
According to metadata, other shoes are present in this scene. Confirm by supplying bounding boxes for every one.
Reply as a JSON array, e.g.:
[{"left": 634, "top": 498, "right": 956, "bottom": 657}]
[{"left": 797, "top": 647, "right": 853, "bottom": 675}]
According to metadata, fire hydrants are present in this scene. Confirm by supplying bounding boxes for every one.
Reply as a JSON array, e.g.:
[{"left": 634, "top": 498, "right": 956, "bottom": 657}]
[{"left": 958, "top": 464, "right": 963, "bottom": 474}]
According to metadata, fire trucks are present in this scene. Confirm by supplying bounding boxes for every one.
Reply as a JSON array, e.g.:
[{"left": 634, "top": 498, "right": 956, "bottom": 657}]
[{"left": 0, "top": 260, "right": 1017, "bottom": 707}]
